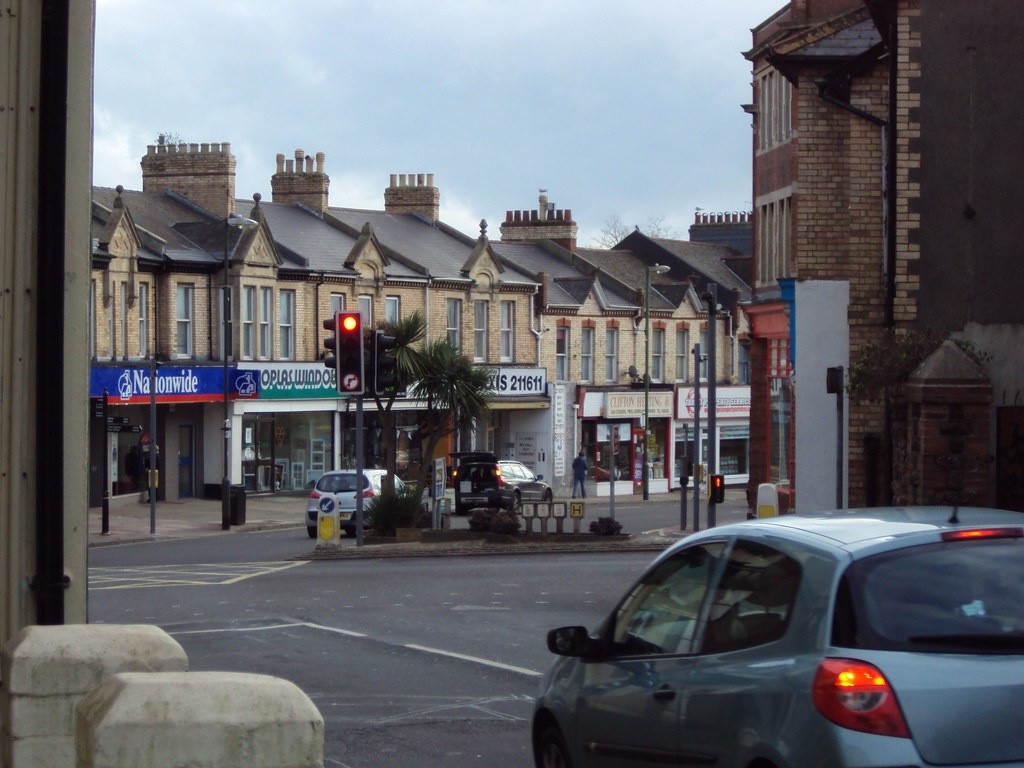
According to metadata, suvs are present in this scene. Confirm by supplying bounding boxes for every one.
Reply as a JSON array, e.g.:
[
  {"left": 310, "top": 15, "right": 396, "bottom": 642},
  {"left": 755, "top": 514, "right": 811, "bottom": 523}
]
[{"left": 448, "top": 450, "right": 554, "bottom": 514}]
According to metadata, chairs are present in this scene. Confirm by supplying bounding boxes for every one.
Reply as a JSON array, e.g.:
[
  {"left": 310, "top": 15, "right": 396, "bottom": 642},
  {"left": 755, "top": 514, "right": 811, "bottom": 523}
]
[
  {"left": 472, "top": 472, "right": 481, "bottom": 480},
  {"left": 337, "top": 479, "right": 349, "bottom": 488},
  {"left": 704, "top": 551, "right": 782, "bottom": 650}
]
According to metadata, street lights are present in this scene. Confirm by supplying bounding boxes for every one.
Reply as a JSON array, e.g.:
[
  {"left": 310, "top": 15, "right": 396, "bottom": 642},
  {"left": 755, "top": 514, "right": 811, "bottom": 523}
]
[
  {"left": 642, "top": 264, "right": 673, "bottom": 500},
  {"left": 701, "top": 281, "right": 723, "bottom": 532},
  {"left": 220, "top": 212, "right": 260, "bottom": 531}
]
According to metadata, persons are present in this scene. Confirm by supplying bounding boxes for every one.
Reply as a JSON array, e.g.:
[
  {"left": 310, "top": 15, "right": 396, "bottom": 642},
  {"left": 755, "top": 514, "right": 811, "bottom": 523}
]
[
  {"left": 124, "top": 444, "right": 159, "bottom": 503},
  {"left": 572, "top": 452, "right": 588, "bottom": 499}
]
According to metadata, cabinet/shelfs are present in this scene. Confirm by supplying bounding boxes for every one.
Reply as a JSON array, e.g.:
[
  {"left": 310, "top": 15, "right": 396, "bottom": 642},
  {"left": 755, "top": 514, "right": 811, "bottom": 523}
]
[
  {"left": 291, "top": 462, "right": 305, "bottom": 489},
  {"left": 312, "top": 439, "right": 325, "bottom": 470}
]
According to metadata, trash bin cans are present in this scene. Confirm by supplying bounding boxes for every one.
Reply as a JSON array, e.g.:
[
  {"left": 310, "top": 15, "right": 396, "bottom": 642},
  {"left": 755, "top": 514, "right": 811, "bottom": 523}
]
[{"left": 229, "top": 483, "right": 246, "bottom": 526}]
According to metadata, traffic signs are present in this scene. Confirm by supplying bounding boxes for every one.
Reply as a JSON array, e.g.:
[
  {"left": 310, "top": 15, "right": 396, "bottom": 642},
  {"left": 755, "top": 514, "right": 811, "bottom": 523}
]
[
  {"left": 107, "top": 424, "right": 142, "bottom": 433},
  {"left": 107, "top": 414, "right": 130, "bottom": 424},
  {"left": 89, "top": 405, "right": 103, "bottom": 411},
  {"left": 89, "top": 412, "right": 104, "bottom": 419},
  {"left": 89, "top": 398, "right": 103, "bottom": 405}
]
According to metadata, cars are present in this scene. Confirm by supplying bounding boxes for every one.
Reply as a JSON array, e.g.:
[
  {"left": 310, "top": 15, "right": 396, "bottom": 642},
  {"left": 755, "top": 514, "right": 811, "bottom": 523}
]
[
  {"left": 531, "top": 508, "right": 1023, "bottom": 768},
  {"left": 304, "top": 470, "right": 409, "bottom": 538}
]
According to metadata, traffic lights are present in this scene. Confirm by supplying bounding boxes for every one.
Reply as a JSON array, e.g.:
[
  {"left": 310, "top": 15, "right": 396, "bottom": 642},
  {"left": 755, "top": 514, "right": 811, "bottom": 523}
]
[
  {"left": 373, "top": 329, "right": 399, "bottom": 397},
  {"left": 335, "top": 309, "right": 367, "bottom": 396},
  {"left": 322, "top": 310, "right": 340, "bottom": 392},
  {"left": 707, "top": 473, "right": 726, "bottom": 505},
  {"left": 363, "top": 327, "right": 382, "bottom": 396}
]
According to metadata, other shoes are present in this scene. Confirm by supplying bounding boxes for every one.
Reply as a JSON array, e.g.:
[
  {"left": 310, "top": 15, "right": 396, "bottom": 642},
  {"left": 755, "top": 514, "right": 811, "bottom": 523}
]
[
  {"left": 572, "top": 496, "right": 576, "bottom": 499},
  {"left": 583, "top": 495, "right": 587, "bottom": 498}
]
